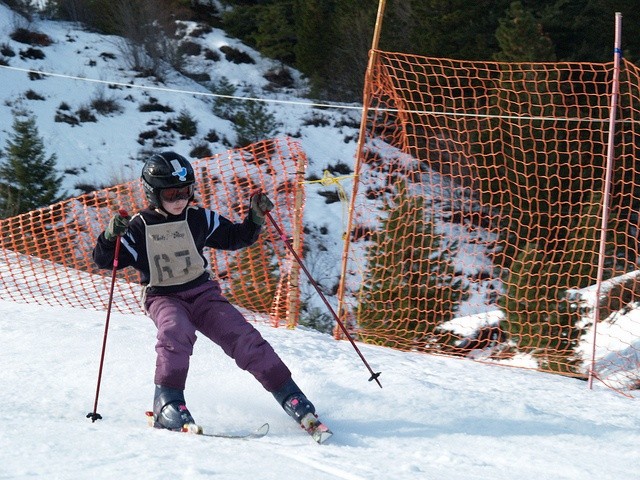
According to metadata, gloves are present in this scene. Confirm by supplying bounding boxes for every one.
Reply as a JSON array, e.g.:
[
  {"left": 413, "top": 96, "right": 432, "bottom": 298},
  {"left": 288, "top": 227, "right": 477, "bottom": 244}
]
[
  {"left": 250, "top": 193, "right": 274, "bottom": 225},
  {"left": 104, "top": 215, "right": 131, "bottom": 241}
]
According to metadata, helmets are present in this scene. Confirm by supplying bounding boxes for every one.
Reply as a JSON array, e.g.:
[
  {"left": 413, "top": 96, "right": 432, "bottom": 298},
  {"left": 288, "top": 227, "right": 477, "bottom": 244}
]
[{"left": 141, "top": 152, "right": 195, "bottom": 209}]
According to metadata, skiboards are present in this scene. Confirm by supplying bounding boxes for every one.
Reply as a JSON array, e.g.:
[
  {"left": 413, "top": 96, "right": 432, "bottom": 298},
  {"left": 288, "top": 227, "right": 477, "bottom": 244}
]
[{"left": 147, "top": 412, "right": 333, "bottom": 443}]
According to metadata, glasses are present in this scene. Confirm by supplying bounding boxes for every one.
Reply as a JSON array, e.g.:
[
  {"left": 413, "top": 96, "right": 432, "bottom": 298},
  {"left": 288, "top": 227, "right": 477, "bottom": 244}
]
[{"left": 159, "top": 184, "right": 194, "bottom": 202}]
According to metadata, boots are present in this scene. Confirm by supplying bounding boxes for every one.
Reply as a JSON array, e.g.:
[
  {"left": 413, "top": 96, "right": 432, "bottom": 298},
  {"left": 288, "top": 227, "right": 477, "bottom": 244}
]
[
  {"left": 272, "top": 378, "right": 315, "bottom": 424},
  {"left": 153, "top": 384, "right": 195, "bottom": 432}
]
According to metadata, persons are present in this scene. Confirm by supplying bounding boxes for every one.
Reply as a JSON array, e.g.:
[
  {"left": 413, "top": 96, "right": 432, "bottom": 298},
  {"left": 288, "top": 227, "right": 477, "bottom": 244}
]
[{"left": 92, "top": 152, "right": 315, "bottom": 429}]
